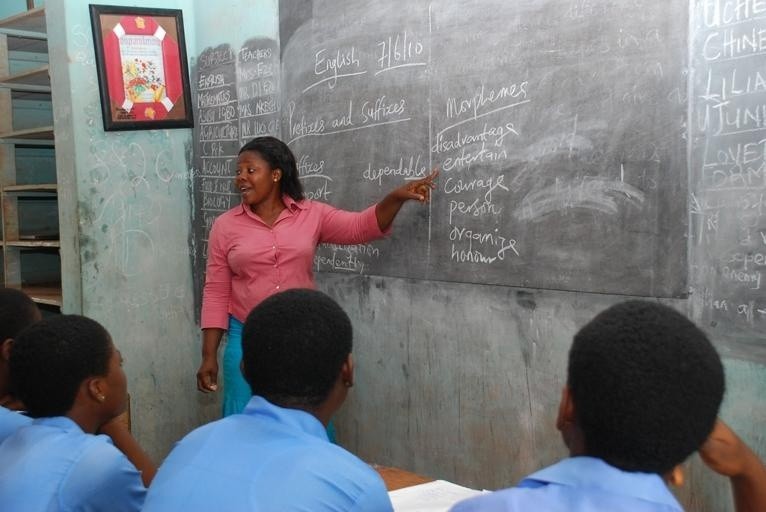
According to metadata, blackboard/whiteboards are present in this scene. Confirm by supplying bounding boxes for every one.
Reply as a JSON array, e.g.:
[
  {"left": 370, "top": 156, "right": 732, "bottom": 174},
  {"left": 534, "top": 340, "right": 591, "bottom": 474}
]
[
  {"left": 687, "top": 1, "right": 766, "bottom": 363},
  {"left": 190, "top": 38, "right": 278, "bottom": 326},
  {"left": 279, "top": 0, "right": 688, "bottom": 298}
]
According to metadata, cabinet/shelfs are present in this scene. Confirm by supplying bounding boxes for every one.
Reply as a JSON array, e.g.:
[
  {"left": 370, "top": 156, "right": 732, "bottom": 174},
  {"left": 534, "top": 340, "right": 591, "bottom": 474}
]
[{"left": 0, "top": 8, "right": 63, "bottom": 307}]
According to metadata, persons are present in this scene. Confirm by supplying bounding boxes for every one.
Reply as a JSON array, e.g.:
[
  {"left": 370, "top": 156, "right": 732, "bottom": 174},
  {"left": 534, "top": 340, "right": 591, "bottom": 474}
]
[
  {"left": 449, "top": 302, "right": 766, "bottom": 512},
  {"left": 0, "top": 289, "right": 42, "bottom": 446},
  {"left": 0, "top": 315, "right": 156, "bottom": 512},
  {"left": 141, "top": 288, "right": 394, "bottom": 512},
  {"left": 196, "top": 137, "right": 438, "bottom": 444}
]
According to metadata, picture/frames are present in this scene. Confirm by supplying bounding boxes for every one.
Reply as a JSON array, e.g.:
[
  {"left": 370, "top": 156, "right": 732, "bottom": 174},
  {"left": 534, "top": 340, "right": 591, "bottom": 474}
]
[{"left": 89, "top": 5, "right": 194, "bottom": 131}]
